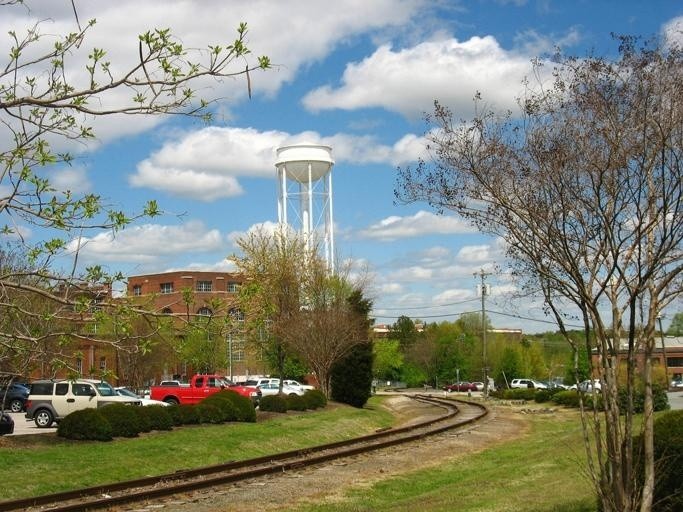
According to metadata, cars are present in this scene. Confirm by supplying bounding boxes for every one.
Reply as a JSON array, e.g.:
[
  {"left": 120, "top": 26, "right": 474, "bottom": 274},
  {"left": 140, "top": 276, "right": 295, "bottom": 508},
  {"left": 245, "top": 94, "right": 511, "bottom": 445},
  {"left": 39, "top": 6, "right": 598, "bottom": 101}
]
[
  {"left": 669, "top": 377, "right": 682, "bottom": 388},
  {"left": 441, "top": 377, "right": 607, "bottom": 394}
]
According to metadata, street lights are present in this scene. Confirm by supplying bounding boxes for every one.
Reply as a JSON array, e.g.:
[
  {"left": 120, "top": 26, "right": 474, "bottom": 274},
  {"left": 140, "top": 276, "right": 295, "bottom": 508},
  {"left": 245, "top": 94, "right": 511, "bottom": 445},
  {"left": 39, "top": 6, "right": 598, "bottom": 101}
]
[
  {"left": 656, "top": 311, "right": 669, "bottom": 390},
  {"left": 225, "top": 324, "right": 233, "bottom": 381}
]
